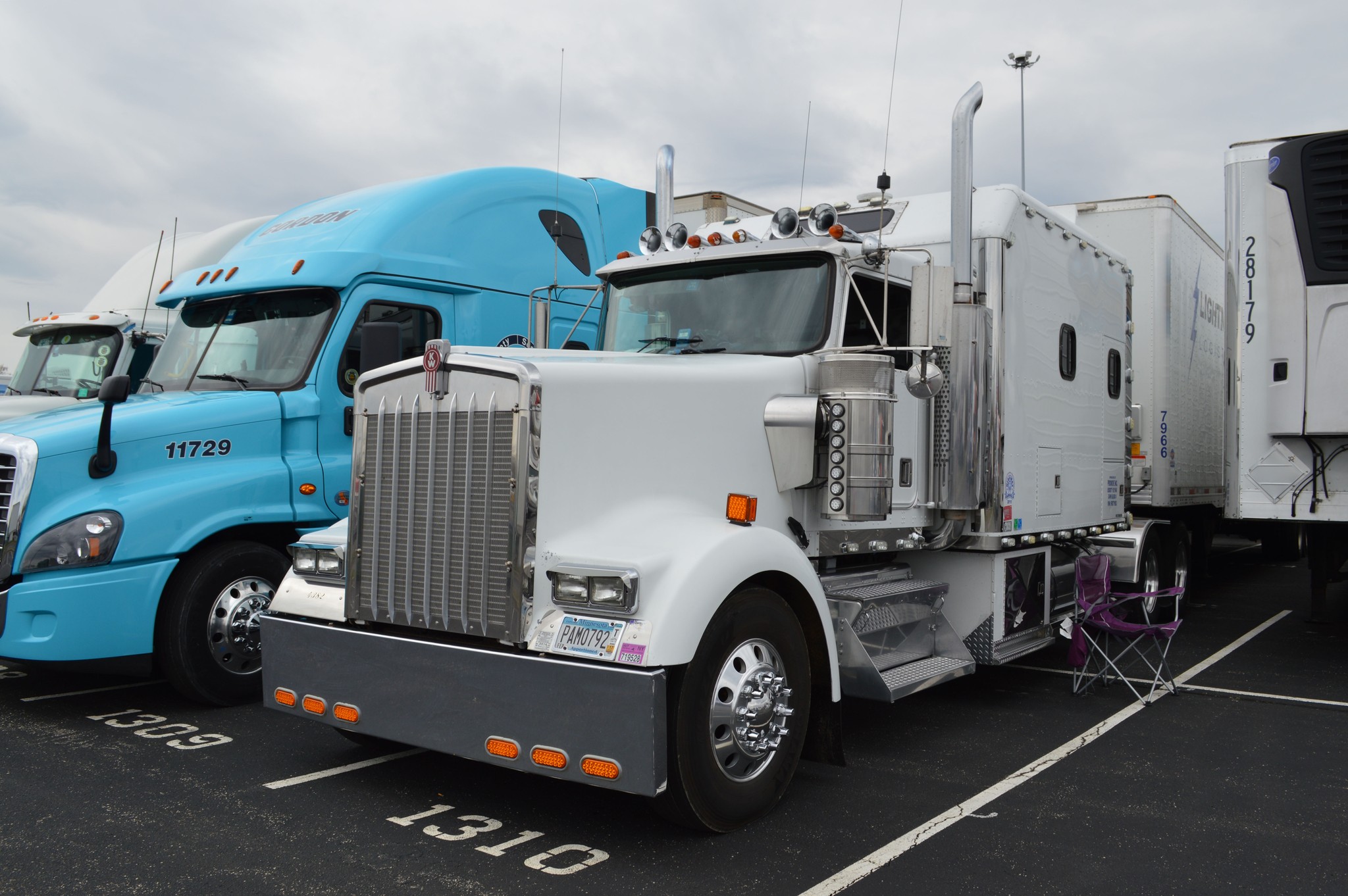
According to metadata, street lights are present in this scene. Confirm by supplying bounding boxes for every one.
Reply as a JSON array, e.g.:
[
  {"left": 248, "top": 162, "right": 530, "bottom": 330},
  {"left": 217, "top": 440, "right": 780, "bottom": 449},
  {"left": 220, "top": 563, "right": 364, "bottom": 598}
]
[{"left": 1002, "top": 50, "right": 1041, "bottom": 191}]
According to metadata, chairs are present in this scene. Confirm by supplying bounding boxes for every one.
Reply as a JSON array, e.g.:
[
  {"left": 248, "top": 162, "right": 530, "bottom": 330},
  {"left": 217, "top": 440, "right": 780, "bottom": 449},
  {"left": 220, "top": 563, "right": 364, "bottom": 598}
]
[{"left": 1074, "top": 554, "right": 1184, "bottom": 706}]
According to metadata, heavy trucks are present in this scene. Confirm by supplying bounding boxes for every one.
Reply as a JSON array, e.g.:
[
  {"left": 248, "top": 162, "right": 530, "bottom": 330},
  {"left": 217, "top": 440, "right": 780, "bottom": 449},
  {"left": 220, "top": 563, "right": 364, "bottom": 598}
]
[
  {"left": 1, "top": 167, "right": 655, "bottom": 707},
  {"left": 260, "top": 0, "right": 1225, "bottom": 832},
  {"left": 0, "top": 216, "right": 264, "bottom": 421}
]
